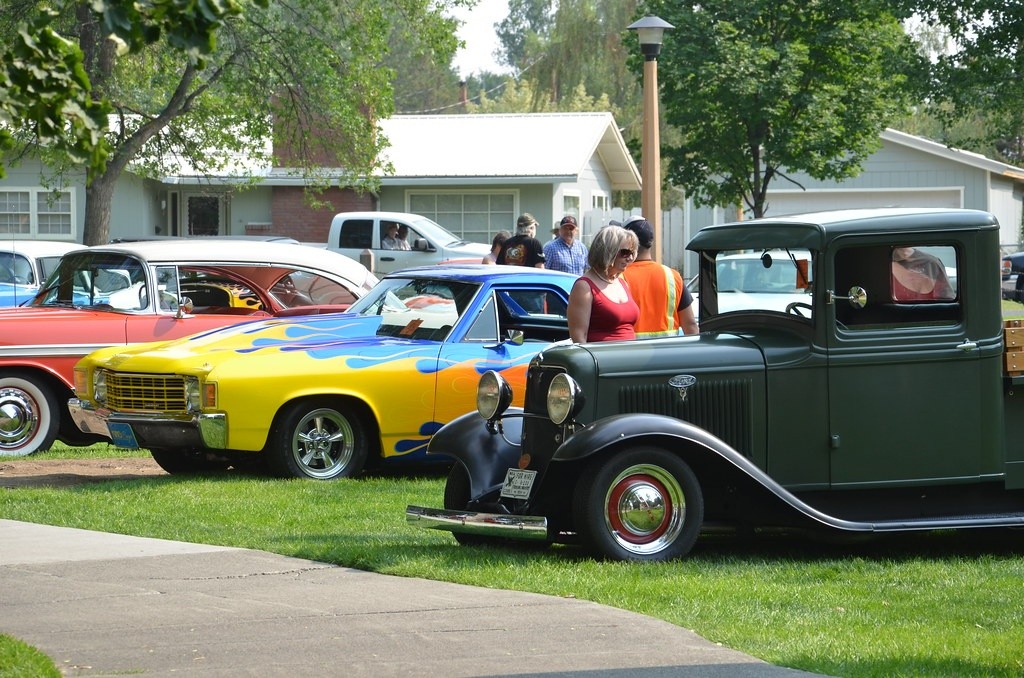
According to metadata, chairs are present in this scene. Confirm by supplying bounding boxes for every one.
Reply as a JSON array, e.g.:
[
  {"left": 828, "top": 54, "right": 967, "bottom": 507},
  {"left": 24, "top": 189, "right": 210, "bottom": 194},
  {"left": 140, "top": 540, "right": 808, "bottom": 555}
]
[{"left": 397, "top": 226, "right": 409, "bottom": 246}]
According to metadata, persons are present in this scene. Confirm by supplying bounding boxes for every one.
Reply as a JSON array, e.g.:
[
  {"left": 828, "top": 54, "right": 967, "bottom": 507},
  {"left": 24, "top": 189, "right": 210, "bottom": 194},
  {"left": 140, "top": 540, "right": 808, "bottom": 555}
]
[
  {"left": 892, "top": 246, "right": 957, "bottom": 299},
  {"left": 542, "top": 215, "right": 588, "bottom": 314},
  {"left": 609, "top": 215, "right": 698, "bottom": 340},
  {"left": 380, "top": 221, "right": 401, "bottom": 250},
  {"left": 540, "top": 221, "right": 561, "bottom": 313},
  {"left": 397, "top": 224, "right": 412, "bottom": 250},
  {"left": 483, "top": 231, "right": 512, "bottom": 265},
  {"left": 568, "top": 225, "right": 640, "bottom": 343},
  {"left": 495, "top": 213, "right": 547, "bottom": 313}
]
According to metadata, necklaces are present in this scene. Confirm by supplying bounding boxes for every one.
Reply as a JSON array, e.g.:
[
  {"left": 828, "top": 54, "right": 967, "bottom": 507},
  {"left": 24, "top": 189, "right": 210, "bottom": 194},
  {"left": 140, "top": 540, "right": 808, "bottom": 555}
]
[{"left": 593, "top": 265, "right": 616, "bottom": 285}]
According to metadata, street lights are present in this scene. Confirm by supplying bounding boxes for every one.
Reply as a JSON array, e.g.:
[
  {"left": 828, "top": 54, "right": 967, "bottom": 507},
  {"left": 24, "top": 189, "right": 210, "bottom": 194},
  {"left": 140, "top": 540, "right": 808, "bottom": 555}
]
[{"left": 623, "top": 12, "right": 678, "bottom": 266}]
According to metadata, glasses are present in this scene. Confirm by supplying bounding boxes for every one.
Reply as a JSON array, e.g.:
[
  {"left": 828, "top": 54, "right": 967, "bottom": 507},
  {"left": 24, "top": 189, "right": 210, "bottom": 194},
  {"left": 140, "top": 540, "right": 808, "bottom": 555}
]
[{"left": 618, "top": 248, "right": 634, "bottom": 258}]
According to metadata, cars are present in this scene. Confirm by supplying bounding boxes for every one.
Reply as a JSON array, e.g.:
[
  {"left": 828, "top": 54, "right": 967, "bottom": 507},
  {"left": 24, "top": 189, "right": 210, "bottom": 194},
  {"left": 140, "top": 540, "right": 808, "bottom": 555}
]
[
  {"left": 676, "top": 248, "right": 818, "bottom": 334},
  {"left": 0, "top": 238, "right": 436, "bottom": 460},
  {"left": 0, "top": 240, "right": 128, "bottom": 312},
  {"left": 66, "top": 263, "right": 583, "bottom": 484}
]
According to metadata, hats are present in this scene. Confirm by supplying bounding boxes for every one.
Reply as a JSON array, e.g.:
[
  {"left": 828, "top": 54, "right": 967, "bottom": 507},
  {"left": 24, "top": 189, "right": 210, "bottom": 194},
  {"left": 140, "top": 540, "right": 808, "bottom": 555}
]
[
  {"left": 609, "top": 215, "right": 653, "bottom": 244},
  {"left": 517, "top": 213, "right": 539, "bottom": 227},
  {"left": 560, "top": 216, "right": 577, "bottom": 228},
  {"left": 551, "top": 221, "right": 561, "bottom": 234}
]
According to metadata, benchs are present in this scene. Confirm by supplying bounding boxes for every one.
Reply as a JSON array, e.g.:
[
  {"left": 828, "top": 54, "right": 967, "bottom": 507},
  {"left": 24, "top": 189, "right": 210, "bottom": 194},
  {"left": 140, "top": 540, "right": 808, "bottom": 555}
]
[
  {"left": 868, "top": 302, "right": 964, "bottom": 324},
  {"left": 191, "top": 306, "right": 270, "bottom": 316}
]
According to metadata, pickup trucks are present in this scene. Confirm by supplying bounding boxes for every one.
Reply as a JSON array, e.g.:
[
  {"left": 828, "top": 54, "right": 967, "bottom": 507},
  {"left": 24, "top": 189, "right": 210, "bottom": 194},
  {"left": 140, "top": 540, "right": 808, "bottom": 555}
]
[
  {"left": 404, "top": 207, "right": 1024, "bottom": 566},
  {"left": 293, "top": 210, "right": 497, "bottom": 286}
]
